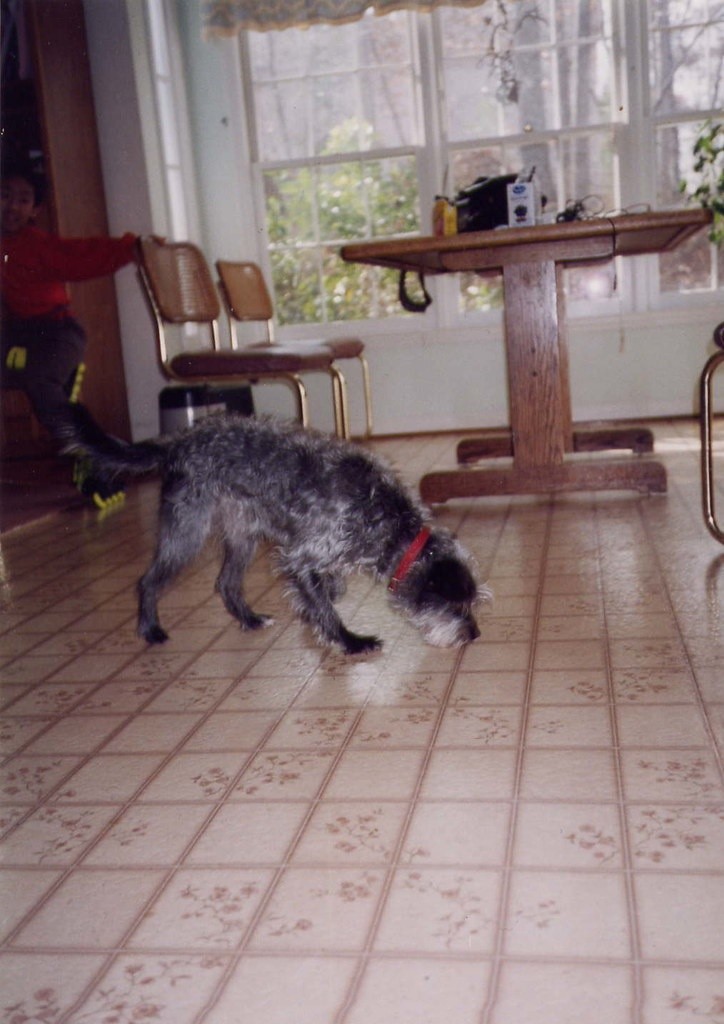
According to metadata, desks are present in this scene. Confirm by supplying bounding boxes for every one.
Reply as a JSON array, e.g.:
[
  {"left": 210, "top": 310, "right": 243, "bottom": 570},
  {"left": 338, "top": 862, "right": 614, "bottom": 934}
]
[{"left": 339, "top": 208, "right": 714, "bottom": 503}]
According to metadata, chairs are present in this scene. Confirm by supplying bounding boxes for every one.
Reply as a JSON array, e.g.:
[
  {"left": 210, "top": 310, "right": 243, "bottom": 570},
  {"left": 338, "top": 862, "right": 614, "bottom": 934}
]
[
  {"left": 216, "top": 259, "right": 372, "bottom": 443},
  {"left": 133, "top": 235, "right": 349, "bottom": 440}
]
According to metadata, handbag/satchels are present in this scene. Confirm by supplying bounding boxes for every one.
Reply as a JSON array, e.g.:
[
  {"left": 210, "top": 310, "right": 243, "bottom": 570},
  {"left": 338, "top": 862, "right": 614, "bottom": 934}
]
[{"left": 453, "top": 173, "right": 547, "bottom": 233}]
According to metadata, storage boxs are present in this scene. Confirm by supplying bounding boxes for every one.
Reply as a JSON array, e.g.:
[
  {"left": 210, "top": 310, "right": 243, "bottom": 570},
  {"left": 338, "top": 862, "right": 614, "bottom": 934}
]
[{"left": 432, "top": 199, "right": 457, "bottom": 234}]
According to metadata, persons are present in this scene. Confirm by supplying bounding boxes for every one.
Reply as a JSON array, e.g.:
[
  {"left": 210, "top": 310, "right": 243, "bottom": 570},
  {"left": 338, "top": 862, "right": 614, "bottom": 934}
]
[{"left": 0, "top": 162, "right": 165, "bottom": 507}]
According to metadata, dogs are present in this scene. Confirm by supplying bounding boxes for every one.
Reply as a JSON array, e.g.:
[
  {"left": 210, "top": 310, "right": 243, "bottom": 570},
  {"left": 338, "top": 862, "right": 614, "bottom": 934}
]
[{"left": 23, "top": 315, "right": 482, "bottom": 658}]
